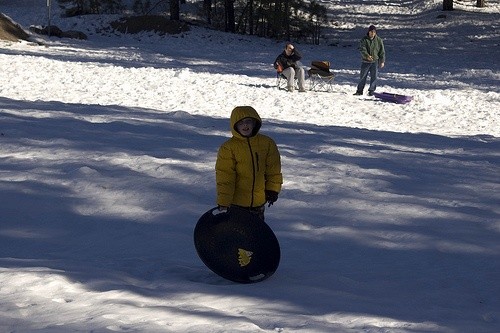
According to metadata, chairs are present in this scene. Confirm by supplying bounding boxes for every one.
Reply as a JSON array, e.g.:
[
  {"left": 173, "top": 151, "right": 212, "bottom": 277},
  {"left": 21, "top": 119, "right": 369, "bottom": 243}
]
[
  {"left": 309, "top": 60, "right": 336, "bottom": 92},
  {"left": 276, "top": 71, "right": 299, "bottom": 91}
]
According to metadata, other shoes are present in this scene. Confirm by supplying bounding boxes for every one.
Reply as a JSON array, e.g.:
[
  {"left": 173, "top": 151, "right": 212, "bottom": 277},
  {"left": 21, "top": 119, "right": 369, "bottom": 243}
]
[
  {"left": 288, "top": 89, "right": 293, "bottom": 93},
  {"left": 353, "top": 92, "right": 363, "bottom": 96},
  {"left": 298, "top": 89, "right": 306, "bottom": 93},
  {"left": 369, "top": 92, "right": 373, "bottom": 96}
]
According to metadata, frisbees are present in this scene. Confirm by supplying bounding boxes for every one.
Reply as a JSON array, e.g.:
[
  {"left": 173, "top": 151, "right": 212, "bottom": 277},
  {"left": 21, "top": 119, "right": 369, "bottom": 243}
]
[{"left": 192, "top": 205, "right": 282, "bottom": 287}]
